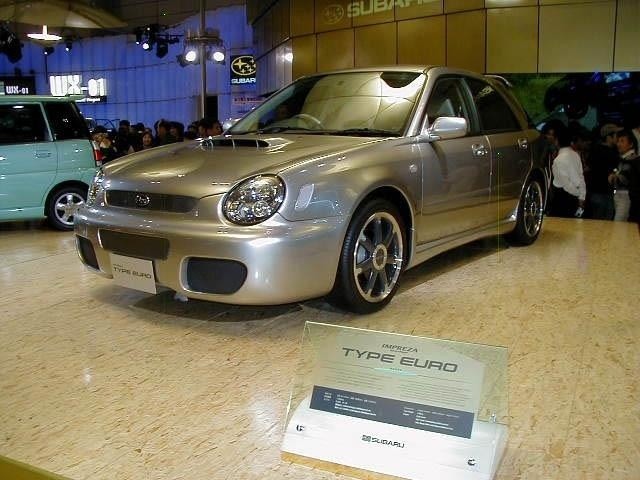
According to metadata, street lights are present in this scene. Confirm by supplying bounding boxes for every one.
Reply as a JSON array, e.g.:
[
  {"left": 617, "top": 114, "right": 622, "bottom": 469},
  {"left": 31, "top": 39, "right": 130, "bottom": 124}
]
[{"left": 180, "top": 38, "right": 228, "bottom": 121}]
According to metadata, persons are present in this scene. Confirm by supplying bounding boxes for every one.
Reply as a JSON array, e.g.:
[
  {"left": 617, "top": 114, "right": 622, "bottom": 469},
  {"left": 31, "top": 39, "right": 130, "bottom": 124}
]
[
  {"left": 88, "top": 116, "right": 224, "bottom": 165},
  {"left": 542, "top": 120, "right": 639, "bottom": 224},
  {"left": 44, "top": 110, "right": 65, "bottom": 140}
]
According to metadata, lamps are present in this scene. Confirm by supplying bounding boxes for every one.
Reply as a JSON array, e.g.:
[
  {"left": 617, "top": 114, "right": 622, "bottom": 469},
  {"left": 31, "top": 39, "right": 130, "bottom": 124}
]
[
  {"left": 127, "top": 20, "right": 180, "bottom": 59},
  {"left": 174, "top": 38, "right": 230, "bottom": 68},
  {"left": 1, "top": 18, "right": 74, "bottom": 62}
]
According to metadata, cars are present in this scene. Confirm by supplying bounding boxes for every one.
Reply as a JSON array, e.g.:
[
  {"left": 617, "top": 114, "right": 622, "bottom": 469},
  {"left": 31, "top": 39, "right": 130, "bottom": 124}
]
[
  {"left": 71, "top": 65, "right": 562, "bottom": 313},
  {"left": 0, "top": 91, "right": 102, "bottom": 234}
]
